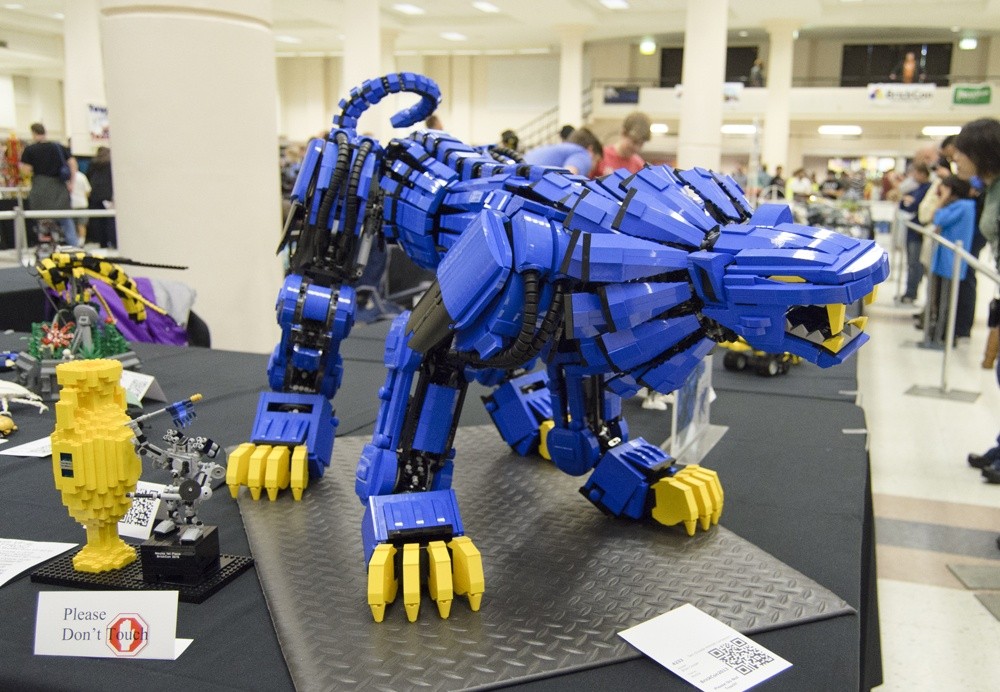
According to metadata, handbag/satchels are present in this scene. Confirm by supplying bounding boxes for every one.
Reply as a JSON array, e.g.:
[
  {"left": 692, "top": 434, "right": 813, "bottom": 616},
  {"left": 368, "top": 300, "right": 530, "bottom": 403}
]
[{"left": 56, "top": 144, "right": 71, "bottom": 180}]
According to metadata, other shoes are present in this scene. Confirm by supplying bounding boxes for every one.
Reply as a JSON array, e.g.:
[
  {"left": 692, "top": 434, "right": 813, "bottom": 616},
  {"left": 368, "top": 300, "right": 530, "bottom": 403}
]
[
  {"left": 984, "top": 461, "right": 1000, "bottom": 483},
  {"left": 969, "top": 448, "right": 1000, "bottom": 469},
  {"left": 916, "top": 323, "right": 927, "bottom": 329},
  {"left": 915, "top": 312, "right": 925, "bottom": 319}
]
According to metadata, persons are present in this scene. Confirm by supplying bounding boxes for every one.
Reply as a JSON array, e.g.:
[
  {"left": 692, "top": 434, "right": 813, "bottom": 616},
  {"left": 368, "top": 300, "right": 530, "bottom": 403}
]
[
  {"left": 733, "top": 119, "right": 1000, "bottom": 484},
  {"left": 523, "top": 109, "right": 651, "bottom": 179},
  {"left": 502, "top": 130, "right": 518, "bottom": 151},
  {"left": 18, "top": 124, "right": 116, "bottom": 248},
  {"left": 890, "top": 50, "right": 927, "bottom": 84},
  {"left": 426, "top": 115, "right": 442, "bottom": 130},
  {"left": 738, "top": 58, "right": 764, "bottom": 87}
]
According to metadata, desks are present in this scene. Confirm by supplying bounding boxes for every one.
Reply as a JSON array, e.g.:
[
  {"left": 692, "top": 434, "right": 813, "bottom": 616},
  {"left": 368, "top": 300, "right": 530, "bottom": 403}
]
[{"left": 1, "top": 264, "right": 885, "bottom": 692}]
